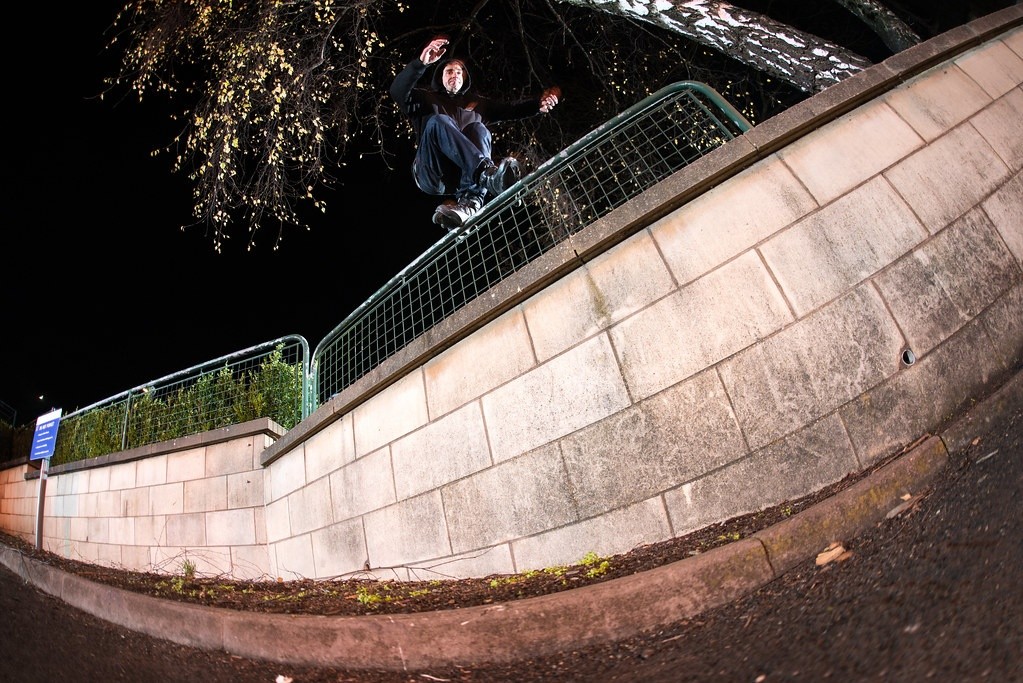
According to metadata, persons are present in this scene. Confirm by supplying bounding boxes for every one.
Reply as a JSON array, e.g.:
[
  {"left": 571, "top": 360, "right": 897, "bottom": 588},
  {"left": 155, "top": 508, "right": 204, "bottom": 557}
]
[{"left": 389, "top": 40, "right": 560, "bottom": 240}]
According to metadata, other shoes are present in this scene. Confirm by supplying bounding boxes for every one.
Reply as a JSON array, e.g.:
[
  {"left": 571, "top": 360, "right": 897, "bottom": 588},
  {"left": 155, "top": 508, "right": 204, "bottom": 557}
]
[
  {"left": 479, "top": 157, "right": 522, "bottom": 207},
  {"left": 432, "top": 204, "right": 475, "bottom": 243}
]
